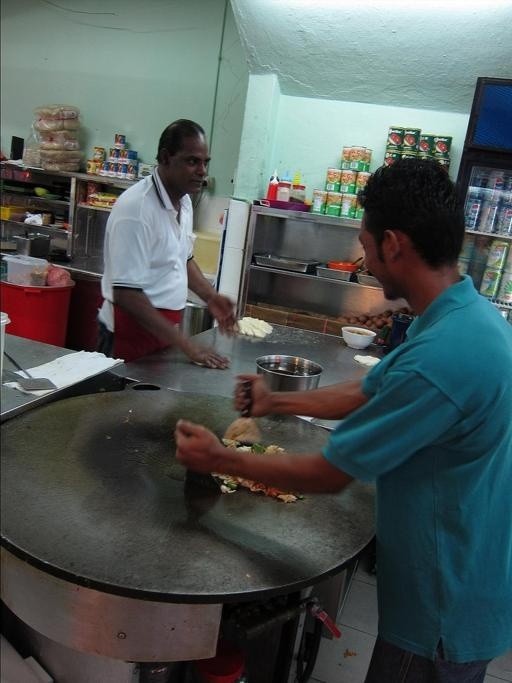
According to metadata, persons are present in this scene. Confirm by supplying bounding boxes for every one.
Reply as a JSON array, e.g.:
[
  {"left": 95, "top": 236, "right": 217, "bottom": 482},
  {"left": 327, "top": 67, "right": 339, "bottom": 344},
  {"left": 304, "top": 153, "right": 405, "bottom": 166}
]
[
  {"left": 174, "top": 159, "right": 512, "bottom": 683},
  {"left": 96, "top": 119, "right": 241, "bottom": 369}
]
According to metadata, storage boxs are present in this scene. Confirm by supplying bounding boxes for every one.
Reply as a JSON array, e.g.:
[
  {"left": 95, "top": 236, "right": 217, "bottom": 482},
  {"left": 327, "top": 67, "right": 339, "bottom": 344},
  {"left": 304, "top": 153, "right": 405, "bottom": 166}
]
[{"left": 1, "top": 254, "right": 48, "bottom": 287}]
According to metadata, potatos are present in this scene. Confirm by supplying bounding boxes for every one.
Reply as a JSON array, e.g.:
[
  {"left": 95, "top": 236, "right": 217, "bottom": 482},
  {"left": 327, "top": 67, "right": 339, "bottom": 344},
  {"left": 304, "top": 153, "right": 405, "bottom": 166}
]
[{"left": 337, "top": 308, "right": 408, "bottom": 329}]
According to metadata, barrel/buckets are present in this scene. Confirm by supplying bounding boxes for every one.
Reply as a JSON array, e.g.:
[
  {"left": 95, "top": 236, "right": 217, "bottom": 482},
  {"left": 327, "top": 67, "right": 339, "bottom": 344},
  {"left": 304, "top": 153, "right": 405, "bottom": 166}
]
[
  {"left": 0, "top": 278, "right": 75, "bottom": 347},
  {"left": 200, "top": 644, "right": 247, "bottom": 683},
  {"left": 0, "top": 307, "right": 11, "bottom": 386}
]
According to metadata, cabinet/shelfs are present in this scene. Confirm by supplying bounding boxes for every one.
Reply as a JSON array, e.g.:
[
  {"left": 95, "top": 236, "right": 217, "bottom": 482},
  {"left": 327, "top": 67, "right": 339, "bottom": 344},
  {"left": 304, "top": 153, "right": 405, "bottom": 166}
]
[
  {"left": 64, "top": 274, "right": 104, "bottom": 361},
  {"left": 451, "top": 76, "right": 512, "bottom": 326},
  {"left": 0, "top": 161, "right": 143, "bottom": 282},
  {"left": 236, "top": 206, "right": 411, "bottom": 348}
]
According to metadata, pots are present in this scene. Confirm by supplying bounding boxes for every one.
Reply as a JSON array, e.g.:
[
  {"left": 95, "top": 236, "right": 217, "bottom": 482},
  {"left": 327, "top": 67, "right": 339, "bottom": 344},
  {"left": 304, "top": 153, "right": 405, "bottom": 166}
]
[
  {"left": 181, "top": 300, "right": 215, "bottom": 341},
  {"left": 0, "top": 390, "right": 376, "bottom": 532},
  {"left": 21, "top": 120, "right": 42, "bottom": 168}
]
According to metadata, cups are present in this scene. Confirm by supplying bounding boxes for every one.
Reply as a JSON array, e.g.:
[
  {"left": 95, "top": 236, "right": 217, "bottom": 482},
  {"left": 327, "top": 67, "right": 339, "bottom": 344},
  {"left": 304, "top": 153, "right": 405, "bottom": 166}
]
[{"left": 255, "top": 354, "right": 324, "bottom": 393}]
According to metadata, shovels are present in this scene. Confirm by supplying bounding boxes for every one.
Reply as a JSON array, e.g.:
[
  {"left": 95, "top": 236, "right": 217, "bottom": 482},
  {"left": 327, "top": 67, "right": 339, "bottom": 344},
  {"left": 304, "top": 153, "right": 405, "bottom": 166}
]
[
  {"left": 223, "top": 381, "right": 263, "bottom": 444},
  {"left": 186, "top": 432, "right": 222, "bottom": 494}
]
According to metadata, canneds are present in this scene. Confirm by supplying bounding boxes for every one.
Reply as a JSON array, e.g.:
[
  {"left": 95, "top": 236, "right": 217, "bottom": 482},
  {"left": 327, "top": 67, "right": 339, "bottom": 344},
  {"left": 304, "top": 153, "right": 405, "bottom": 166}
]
[
  {"left": 86, "top": 133, "right": 138, "bottom": 181},
  {"left": 456, "top": 168, "right": 512, "bottom": 322},
  {"left": 309, "top": 146, "right": 374, "bottom": 220},
  {"left": 383, "top": 126, "right": 452, "bottom": 170}
]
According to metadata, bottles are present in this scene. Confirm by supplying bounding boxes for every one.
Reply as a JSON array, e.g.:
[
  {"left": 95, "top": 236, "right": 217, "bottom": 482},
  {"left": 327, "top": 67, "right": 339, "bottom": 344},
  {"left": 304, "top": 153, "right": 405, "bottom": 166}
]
[
  {"left": 267, "top": 169, "right": 306, "bottom": 205},
  {"left": 41, "top": 210, "right": 65, "bottom": 226}
]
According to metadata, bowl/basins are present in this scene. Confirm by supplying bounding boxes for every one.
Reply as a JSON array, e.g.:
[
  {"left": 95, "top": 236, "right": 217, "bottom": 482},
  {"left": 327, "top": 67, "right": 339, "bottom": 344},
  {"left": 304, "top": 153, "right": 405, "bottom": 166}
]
[
  {"left": 341, "top": 326, "right": 377, "bottom": 350},
  {"left": 328, "top": 260, "right": 360, "bottom": 272}
]
